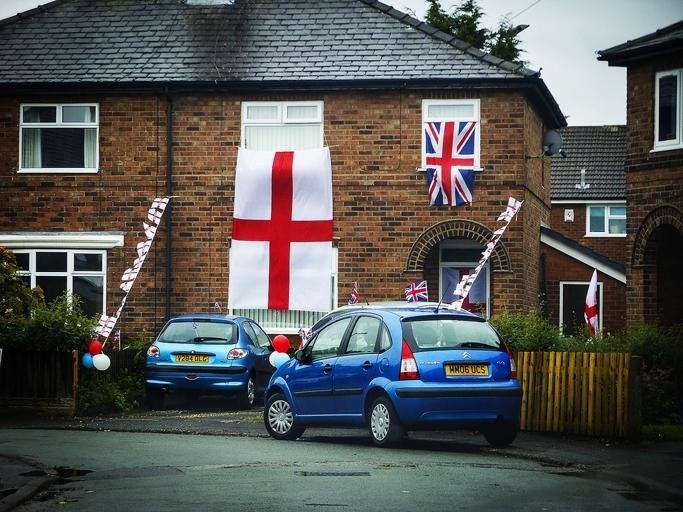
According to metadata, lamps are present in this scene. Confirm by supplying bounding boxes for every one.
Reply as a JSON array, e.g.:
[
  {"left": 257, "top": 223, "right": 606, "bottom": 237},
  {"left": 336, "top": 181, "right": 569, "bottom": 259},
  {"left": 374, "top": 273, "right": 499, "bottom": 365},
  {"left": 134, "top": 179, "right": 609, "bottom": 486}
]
[{"left": 526, "top": 128, "right": 563, "bottom": 159}]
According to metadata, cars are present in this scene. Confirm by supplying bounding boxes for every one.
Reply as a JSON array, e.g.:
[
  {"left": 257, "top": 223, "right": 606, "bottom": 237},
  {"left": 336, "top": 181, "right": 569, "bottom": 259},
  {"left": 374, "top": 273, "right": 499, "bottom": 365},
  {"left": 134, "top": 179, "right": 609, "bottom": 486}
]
[
  {"left": 263, "top": 299, "right": 524, "bottom": 451},
  {"left": 143, "top": 312, "right": 275, "bottom": 409}
]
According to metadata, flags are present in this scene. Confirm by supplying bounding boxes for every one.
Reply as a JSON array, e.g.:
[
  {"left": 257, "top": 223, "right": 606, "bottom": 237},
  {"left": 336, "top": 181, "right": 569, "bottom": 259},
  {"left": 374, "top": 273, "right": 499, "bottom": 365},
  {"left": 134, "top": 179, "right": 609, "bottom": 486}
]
[
  {"left": 422, "top": 119, "right": 475, "bottom": 208},
  {"left": 404, "top": 279, "right": 427, "bottom": 303},
  {"left": 583, "top": 266, "right": 601, "bottom": 342},
  {"left": 347, "top": 280, "right": 359, "bottom": 305},
  {"left": 227, "top": 143, "right": 334, "bottom": 314},
  {"left": 93, "top": 197, "right": 168, "bottom": 338},
  {"left": 110, "top": 331, "right": 120, "bottom": 344},
  {"left": 448, "top": 197, "right": 522, "bottom": 316}
]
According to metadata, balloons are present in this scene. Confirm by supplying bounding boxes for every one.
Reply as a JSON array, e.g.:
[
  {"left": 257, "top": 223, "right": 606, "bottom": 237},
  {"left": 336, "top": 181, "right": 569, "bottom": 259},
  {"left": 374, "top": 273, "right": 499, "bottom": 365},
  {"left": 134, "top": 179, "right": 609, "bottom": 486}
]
[
  {"left": 268, "top": 349, "right": 278, "bottom": 365},
  {"left": 82, "top": 352, "right": 93, "bottom": 368},
  {"left": 86, "top": 340, "right": 101, "bottom": 355},
  {"left": 274, "top": 352, "right": 290, "bottom": 369},
  {"left": 90, "top": 353, "right": 110, "bottom": 370},
  {"left": 272, "top": 333, "right": 291, "bottom": 354}
]
